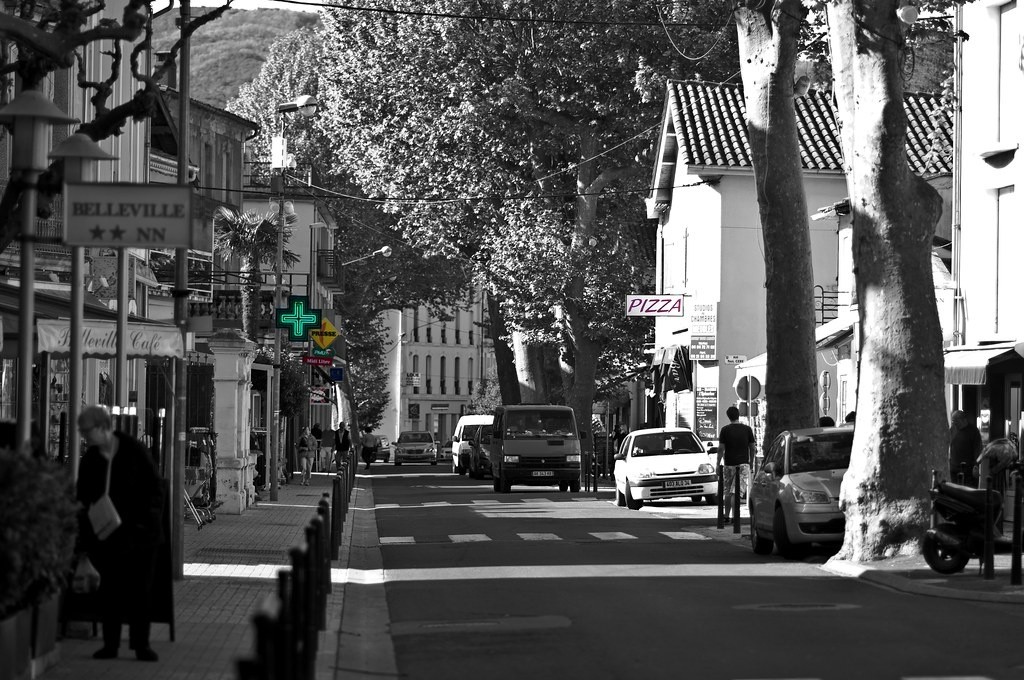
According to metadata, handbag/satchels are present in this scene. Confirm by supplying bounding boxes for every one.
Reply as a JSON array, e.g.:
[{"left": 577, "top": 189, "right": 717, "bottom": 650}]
[
  {"left": 73, "top": 555, "right": 100, "bottom": 594},
  {"left": 88, "top": 495, "right": 122, "bottom": 541}
]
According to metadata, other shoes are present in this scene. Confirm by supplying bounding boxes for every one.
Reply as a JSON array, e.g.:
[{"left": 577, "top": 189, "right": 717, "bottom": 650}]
[
  {"left": 723, "top": 514, "right": 734, "bottom": 526},
  {"left": 305, "top": 483, "right": 309, "bottom": 485},
  {"left": 91, "top": 648, "right": 119, "bottom": 659},
  {"left": 322, "top": 468, "right": 326, "bottom": 472},
  {"left": 300, "top": 481, "right": 304, "bottom": 485},
  {"left": 135, "top": 649, "right": 159, "bottom": 662}
]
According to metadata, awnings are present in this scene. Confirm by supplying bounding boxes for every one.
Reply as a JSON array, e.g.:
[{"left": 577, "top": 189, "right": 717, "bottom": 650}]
[
  {"left": 732, "top": 309, "right": 861, "bottom": 399},
  {"left": 0, "top": 302, "right": 185, "bottom": 361},
  {"left": 945, "top": 348, "right": 1014, "bottom": 385}
]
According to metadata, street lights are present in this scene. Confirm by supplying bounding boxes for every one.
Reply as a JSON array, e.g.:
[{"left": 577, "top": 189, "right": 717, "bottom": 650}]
[
  {"left": 1, "top": 90, "right": 81, "bottom": 460},
  {"left": 269, "top": 94, "right": 321, "bottom": 503},
  {"left": 47, "top": 134, "right": 121, "bottom": 506}
]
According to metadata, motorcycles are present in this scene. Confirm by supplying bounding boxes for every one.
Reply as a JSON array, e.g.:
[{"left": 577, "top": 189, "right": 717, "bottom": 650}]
[{"left": 923, "top": 469, "right": 1024, "bottom": 574}]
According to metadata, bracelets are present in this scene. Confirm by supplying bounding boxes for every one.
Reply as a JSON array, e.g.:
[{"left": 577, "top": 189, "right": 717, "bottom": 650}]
[{"left": 974, "top": 466, "right": 978, "bottom": 470}]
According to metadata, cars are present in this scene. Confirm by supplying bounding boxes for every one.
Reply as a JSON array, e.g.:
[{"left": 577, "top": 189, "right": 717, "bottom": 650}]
[
  {"left": 440, "top": 441, "right": 454, "bottom": 462},
  {"left": 613, "top": 427, "right": 719, "bottom": 510},
  {"left": 391, "top": 430, "right": 441, "bottom": 466},
  {"left": 468, "top": 426, "right": 494, "bottom": 479},
  {"left": 748, "top": 425, "right": 855, "bottom": 559}
]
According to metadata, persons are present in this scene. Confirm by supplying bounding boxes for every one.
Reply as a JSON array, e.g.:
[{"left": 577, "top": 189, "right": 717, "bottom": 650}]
[
  {"left": 949, "top": 410, "right": 983, "bottom": 491},
  {"left": 296, "top": 422, "right": 375, "bottom": 486},
  {"left": 612, "top": 425, "right": 627, "bottom": 454},
  {"left": 76, "top": 404, "right": 167, "bottom": 662},
  {"left": 821, "top": 410, "right": 856, "bottom": 427},
  {"left": 716, "top": 405, "right": 757, "bottom": 524}
]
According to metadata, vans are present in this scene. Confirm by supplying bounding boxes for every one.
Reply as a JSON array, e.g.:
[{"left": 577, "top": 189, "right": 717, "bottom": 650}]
[
  {"left": 480, "top": 404, "right": 587, "bottom": 493},
  {"left": 348, "top": 435, "right": 390, "bottom": 463},
  {"left": 450, "top": 415, "right": 495, "bottom": 476}
]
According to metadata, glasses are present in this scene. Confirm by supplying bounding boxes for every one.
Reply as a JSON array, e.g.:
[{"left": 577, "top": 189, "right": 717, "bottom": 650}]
[{"left": 78, "top": 425, "right": 97, "bottom": 433}]
